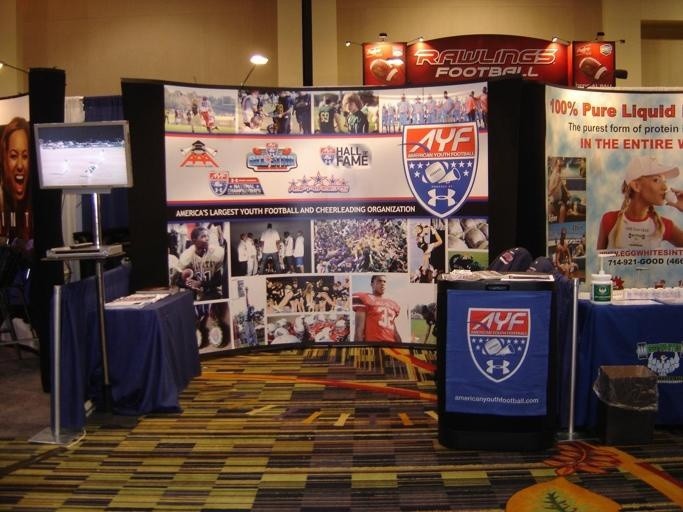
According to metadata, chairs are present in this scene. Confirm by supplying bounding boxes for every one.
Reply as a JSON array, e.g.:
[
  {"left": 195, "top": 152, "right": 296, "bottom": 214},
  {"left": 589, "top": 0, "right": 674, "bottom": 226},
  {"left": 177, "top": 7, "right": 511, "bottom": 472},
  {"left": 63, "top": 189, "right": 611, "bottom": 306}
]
[
  {"left": 525, "top": 254, "right": 553, "bottom": 271},
  {"left": 490, "top": 247, "right": 532, "bottom": 271}
]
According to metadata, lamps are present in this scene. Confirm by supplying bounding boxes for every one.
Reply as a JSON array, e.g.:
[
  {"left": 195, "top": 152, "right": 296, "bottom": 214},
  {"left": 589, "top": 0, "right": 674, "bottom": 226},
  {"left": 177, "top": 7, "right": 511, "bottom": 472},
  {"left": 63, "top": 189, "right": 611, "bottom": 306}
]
[
  {"left": 550, "top": 29, "right": 628, "bottom": 55},
  {"left": 237, "top": 53, "right": 271, "bottom": 88},
  {"left": 344, "top": 33, "right": 434, "bottom": 60}
]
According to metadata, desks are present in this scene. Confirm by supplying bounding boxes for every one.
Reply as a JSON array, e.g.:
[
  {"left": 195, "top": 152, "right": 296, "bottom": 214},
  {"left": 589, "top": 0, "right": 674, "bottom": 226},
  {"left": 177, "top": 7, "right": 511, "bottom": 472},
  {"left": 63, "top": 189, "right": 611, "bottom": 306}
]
[
  {"left": 572, "top": 287, "right": 681, "bottom": 440},
  {"left": 98, "top": 284, "right": 200, "bottom": 417}
]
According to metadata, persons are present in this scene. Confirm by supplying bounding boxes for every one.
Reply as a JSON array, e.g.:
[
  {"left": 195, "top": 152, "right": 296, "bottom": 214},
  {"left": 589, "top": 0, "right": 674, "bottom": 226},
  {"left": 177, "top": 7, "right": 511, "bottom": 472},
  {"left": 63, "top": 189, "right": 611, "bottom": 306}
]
[
  {"left": 382, "top": 86, "right": 487, "bottom": 132},
  {"left": 164, "top": 84, "right": 378, "bottom": 135},
  {"left": 593, "top": 156, "right": 682, "bottom": 249},
  {"left": 548, "top": 159, "right": 570, "bottom": 223},
  {"left": 554, "top": 227, "right": 578, "bottom": 279},
  {"left": 1, "top": 117, "right": 30, "bottom": 208},
  {"left": 168, "top": 219, "right": 442, "bottom": 343}
]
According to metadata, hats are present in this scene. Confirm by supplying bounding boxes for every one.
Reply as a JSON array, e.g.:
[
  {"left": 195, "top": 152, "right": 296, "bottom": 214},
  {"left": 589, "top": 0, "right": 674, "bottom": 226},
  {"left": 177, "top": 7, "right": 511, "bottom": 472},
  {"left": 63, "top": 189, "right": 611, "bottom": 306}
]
[{"left": 623, "top": 157, "right": 680, "bottom": 185}]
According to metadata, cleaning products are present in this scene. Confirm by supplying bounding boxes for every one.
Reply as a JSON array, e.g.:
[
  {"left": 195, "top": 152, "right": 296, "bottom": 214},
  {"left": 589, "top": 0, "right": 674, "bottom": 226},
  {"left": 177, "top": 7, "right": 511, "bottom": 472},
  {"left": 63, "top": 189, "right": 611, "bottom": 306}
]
[{"left": 590, "top": 253, "right": 616, "bottom": 305}]
